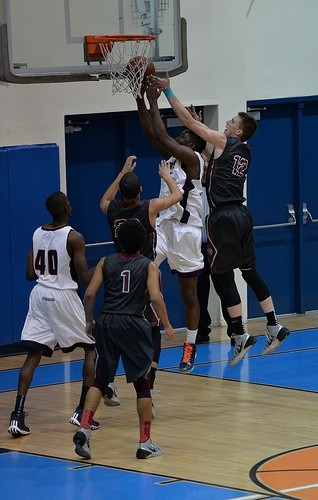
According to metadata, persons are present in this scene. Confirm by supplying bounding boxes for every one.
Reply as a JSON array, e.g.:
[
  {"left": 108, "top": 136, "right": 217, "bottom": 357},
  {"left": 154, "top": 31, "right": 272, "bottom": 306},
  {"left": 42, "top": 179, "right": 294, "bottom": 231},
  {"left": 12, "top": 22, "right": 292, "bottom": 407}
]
[
  {"left": 71, "top": 219, "right": 177, "bottom": 458},
  {"left": 7, "top": 192, "right": 100, "bottom": 438},
  {"left": 101, "top": 71, "right": 291, "bottom": 415}
]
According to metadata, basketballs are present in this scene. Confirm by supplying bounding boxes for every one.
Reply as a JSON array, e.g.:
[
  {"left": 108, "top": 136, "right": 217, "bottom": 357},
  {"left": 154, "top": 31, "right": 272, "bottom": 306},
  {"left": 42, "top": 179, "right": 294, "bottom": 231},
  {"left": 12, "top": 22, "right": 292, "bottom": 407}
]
[{"left": 127, "top": 56, "right": 155, "bottom": 80}]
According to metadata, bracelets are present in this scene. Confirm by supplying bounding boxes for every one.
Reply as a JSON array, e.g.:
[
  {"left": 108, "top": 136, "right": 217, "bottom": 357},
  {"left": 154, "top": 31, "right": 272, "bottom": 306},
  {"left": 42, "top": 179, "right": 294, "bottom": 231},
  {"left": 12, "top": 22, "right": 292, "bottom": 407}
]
[{"left": 163, "top": 87, "right": 175, "bottom": 100}]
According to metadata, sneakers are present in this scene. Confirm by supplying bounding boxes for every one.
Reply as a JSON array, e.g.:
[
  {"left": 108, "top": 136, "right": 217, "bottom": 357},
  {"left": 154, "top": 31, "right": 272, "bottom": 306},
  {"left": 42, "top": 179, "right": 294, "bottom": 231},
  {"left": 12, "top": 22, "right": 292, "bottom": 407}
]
[
  {"left": 136, "top": 438, "right": 162, "bottom": 459},
  {"left": 179, "top": 340, "right": 197, "bottom": 373},
  {"left": 7, "top": 412, "right": 30, "bottom": 435},
  {"left": 230, "top": 333, "right": 257, "bottom": 366},
  {"left": 150, "top": 389, "right": 160, "bottom": 419},
  {"left": 73, "top": 428, "right": 91, "bottom": 458},
  {"left": 102, "top": 384, "right": 121, "bottom": 406},
  {"left": 68, "top": 408, "right": 100, "bottom": 429},
  {"left": 262, "top": 323, "right": 290, "bottom": 355}
]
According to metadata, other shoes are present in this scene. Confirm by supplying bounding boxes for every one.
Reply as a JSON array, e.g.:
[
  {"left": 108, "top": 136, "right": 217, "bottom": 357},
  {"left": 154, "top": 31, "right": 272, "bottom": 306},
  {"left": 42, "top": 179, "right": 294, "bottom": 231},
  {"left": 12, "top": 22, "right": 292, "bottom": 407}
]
[{"left": 195, "top": 336, "right": 210, "bottom": 344}]
